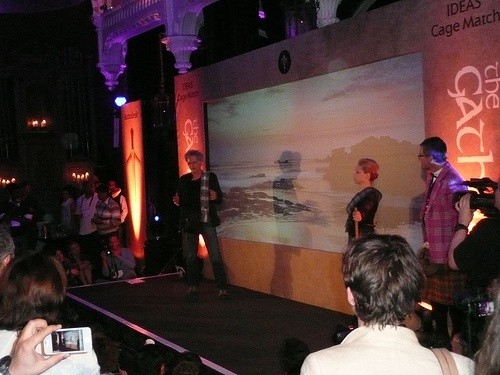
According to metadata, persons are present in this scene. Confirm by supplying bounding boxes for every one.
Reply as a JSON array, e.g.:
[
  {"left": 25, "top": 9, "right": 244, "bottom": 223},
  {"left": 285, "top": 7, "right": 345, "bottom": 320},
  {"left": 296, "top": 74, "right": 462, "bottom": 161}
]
[
  {"left": 173, "top": 149, "right": 234, "bottom": 300},
  {"left": 0, "top": 318, "right": 71, "bottom": 375},
  {"left": 90, "top": 334, "right": 314, "bottom": 375},
  {"left": 343, "top": 157, "right": 382, "bottom": 324},
  {"left": 414, "top": 136, "right": 468, "bottom": 354},
  {"left": 448, "top": 180, "right": 500, "bottom": 356},
  {"left": 0, "top": 172, "right": 138, "bottom": 329},
  {"left": 0, "top": 255, "right": 101, "bottom": 374}
]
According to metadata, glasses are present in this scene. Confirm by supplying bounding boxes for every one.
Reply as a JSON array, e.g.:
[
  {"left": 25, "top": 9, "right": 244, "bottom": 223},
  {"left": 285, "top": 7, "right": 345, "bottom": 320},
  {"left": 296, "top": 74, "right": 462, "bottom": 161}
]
[
  {"left": 187, "top": 160, "right": 200, "bottom": 166},
  {"left": 418, "top": 153, "right": 426, "bottom": 160}
]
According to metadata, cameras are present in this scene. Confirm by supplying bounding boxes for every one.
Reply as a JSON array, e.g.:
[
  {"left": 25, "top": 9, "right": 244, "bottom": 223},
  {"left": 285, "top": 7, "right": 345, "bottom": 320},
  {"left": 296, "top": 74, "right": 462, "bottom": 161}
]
[{"left": 470, "top": 298, "right": 495, "bottom": 317}]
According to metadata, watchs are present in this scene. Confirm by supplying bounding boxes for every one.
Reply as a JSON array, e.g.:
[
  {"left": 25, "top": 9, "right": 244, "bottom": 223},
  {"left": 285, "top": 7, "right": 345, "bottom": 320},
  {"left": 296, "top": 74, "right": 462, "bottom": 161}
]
[
  {"left": 454, "top": 224, "right": 468, "bottom": 235},
  {"left": 0, "top": 355, "right": 12, "bottom": 375}
]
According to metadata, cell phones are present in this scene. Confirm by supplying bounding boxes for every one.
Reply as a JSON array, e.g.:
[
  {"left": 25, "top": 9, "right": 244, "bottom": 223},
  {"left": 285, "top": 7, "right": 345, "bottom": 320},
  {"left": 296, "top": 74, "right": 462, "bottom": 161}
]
[{"left": 43, "top": 327, "right": 92, "bottom": 355}]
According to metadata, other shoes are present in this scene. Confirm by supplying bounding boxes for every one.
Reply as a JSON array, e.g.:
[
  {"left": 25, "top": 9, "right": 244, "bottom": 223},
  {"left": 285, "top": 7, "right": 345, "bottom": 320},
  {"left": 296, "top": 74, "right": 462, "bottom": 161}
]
[{"left": 219, "top": 293, "right": 229, "bottom": 300}]
